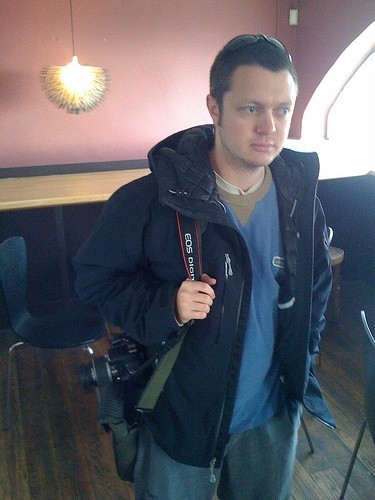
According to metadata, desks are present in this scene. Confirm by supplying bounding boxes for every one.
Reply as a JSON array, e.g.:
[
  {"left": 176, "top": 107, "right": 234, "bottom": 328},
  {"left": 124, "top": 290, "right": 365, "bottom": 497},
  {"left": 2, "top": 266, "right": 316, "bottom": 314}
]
[{"left": 0, "top": 169, "right": 152, "bottom": 301}]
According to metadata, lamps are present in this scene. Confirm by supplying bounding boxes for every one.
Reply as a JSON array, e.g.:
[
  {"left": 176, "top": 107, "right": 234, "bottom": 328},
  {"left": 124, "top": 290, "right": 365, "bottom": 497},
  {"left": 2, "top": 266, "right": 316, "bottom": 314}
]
[{"left": 38, "top": 0, "right": 109, "bottom": 114}]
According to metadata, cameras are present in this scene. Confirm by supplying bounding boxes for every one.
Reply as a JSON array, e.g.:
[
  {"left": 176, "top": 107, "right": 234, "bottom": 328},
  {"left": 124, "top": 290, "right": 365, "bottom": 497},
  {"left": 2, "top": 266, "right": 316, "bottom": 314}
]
[{"left": 82, "top": 335, "right": 149, "bottom": 393}]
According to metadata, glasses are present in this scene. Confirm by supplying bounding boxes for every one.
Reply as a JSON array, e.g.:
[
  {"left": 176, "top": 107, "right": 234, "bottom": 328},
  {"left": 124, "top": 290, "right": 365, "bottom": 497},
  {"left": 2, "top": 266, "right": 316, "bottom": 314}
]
[{"left": 213, "top": 34, "right": 293, "bottom": 69}]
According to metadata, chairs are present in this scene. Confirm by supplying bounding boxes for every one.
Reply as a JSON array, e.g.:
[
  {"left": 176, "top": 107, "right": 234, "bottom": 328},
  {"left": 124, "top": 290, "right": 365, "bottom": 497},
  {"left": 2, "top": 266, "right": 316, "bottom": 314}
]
[
  {"left": 339, "top": 311, "right": 375, "bottom": 500},
  {"left": 0, "top": 237, "right": 107, "bottom": 431}
]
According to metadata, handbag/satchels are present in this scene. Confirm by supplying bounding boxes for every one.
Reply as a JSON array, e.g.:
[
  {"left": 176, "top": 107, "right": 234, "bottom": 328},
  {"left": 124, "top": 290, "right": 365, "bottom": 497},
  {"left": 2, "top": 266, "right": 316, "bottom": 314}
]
[{"left": 96, "top": 376, "right": 141, "bottom": 483}]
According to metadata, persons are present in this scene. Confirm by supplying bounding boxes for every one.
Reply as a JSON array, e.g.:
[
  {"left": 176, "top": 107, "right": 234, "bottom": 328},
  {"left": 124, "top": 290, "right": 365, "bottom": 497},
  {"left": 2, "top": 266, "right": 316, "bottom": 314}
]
[{"left": 72, "top": 33, "right": 337, "bottom": 500}]
